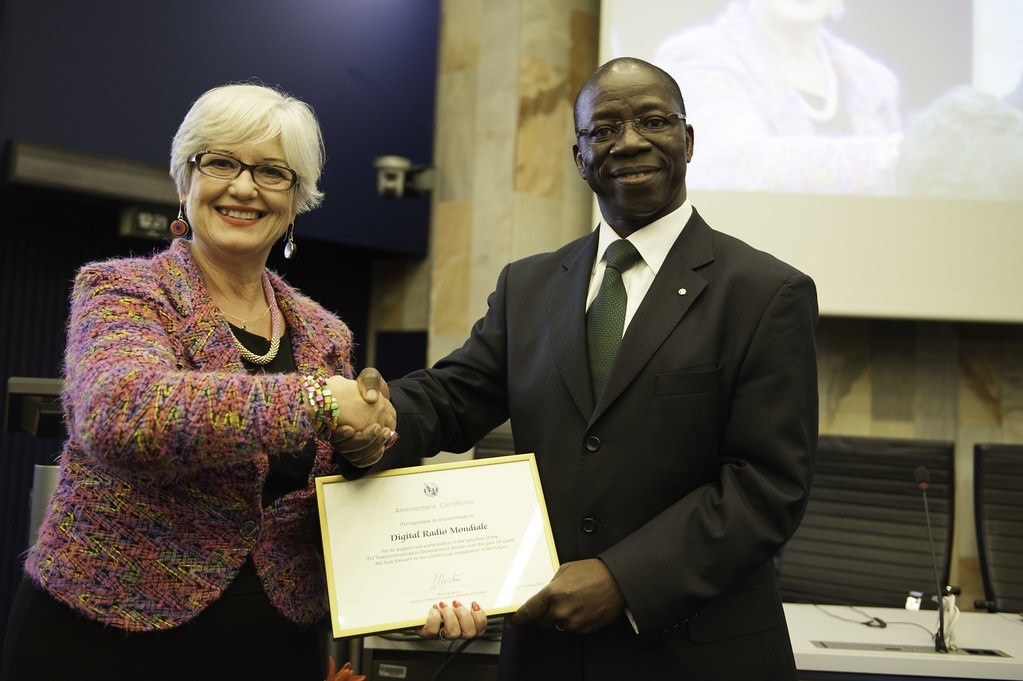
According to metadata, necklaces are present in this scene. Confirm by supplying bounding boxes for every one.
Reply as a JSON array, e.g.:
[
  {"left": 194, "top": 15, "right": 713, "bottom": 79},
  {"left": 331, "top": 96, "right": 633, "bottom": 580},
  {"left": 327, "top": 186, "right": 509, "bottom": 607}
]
[
  {"left": 740, "top": 24, "right": 839, "bottom": 122},
  {"left": 228, "top": 273, "right": 280, "bottom": 366},
  {"left": 219, "top": 305, "right": 272, "bottom": 330}
]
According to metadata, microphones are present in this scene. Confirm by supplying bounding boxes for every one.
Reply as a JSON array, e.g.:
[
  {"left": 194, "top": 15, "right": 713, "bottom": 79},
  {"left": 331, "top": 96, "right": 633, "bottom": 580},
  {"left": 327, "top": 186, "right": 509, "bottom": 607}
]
[{"left": 913, "top": 465, "right": 948, "bottom": 652}]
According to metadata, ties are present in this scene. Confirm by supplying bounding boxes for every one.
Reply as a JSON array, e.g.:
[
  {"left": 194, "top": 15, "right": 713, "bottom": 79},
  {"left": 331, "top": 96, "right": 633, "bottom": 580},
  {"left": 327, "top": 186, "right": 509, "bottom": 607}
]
[{"left": 585, "top": 236, "right": 642, "bottom": 408}]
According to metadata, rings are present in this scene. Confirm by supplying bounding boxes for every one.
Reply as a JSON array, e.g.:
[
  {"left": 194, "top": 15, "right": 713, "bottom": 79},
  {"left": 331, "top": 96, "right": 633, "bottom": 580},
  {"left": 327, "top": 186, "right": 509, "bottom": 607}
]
[
  {"left": 555, "top": 625, "right": 566, "bottom": 632},
  {"left": 439, "top": 629, "right": 446, "bottom": 641}
]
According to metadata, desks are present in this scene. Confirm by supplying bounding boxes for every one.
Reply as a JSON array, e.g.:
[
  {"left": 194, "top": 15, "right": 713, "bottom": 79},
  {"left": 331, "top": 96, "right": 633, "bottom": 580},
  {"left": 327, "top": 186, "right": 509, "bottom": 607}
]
[{"left": 363, "top": 604, "right": 1023, "bottom": 681}]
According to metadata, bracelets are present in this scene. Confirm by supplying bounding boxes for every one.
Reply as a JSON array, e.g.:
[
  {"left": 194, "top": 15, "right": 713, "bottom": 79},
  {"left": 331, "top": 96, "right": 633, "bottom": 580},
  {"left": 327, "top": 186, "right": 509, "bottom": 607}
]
[{"left": 302, "top": 374, "right": 339, "bottom": 440}]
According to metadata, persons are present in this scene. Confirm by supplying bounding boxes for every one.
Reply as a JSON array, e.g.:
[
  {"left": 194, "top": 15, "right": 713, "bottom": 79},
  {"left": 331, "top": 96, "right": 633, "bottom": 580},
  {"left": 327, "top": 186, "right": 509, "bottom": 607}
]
[
  {"left": 650, "top": 0, "right": 900, "bottom": 196},
  {"left": 1, "top": 83, "right": 397, "bottom": 681},
  {"left": 326, "top": 57, "right": 821, "bottom": 681}
]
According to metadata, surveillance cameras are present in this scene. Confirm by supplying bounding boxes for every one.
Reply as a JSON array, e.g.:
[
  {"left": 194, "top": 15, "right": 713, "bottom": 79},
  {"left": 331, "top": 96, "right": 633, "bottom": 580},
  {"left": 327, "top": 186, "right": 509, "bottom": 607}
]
[{"left": 376, "top": 168, "right": 404, "bottom": 200}]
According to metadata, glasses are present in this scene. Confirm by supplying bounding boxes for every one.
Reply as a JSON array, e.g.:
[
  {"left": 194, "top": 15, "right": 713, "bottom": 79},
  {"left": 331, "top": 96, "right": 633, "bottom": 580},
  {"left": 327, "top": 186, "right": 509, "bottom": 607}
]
[
  {"left": 577, "top": 113, "right": 686, "bottom": 143},
  {"left": 187, "top": 150, "right": 301, "bottom": 192}
]
